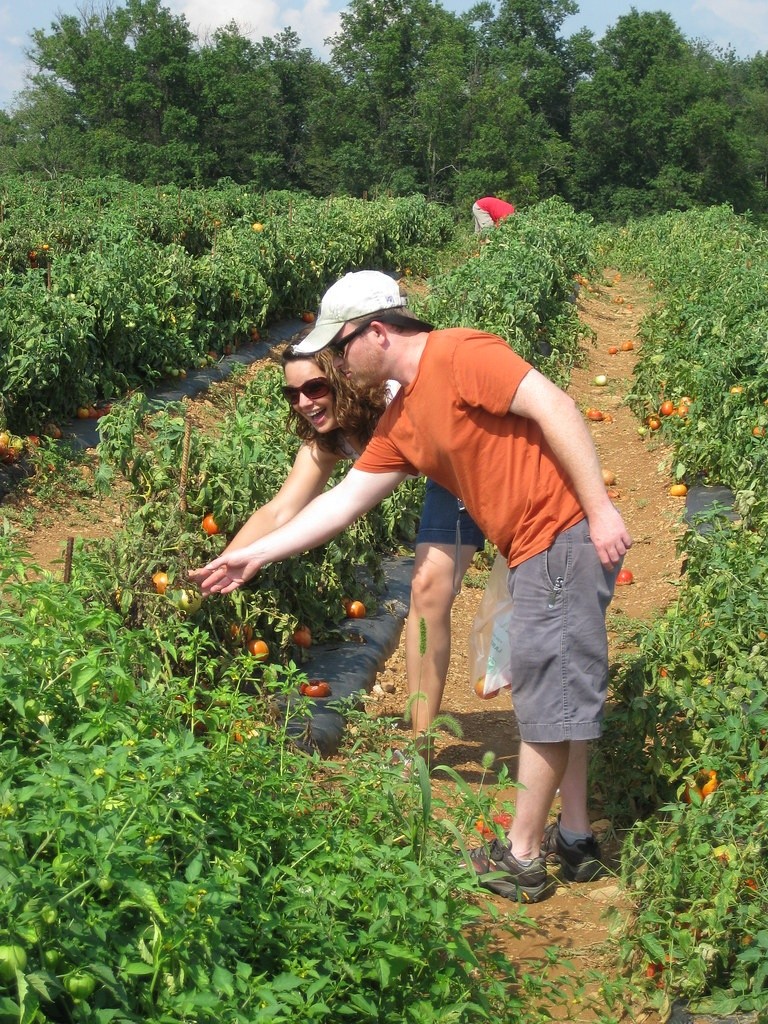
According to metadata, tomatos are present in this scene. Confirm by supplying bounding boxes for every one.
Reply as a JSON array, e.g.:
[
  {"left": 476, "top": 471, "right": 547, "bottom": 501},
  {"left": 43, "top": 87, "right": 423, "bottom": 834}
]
[{"left": 1, "top": 220, "right": 768, "bottom": 1024}]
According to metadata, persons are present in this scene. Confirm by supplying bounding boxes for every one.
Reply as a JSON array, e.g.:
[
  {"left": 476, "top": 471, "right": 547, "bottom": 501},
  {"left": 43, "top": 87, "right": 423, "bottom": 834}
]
[
  {"left": 189, "top": 322, "right": 486, "bottom": 785},
  {"left": 201, "top": 270, "right": 635, "bottom": 907},
  {"left": 472, "top": 198, "right": 515, "bottom": 234}
]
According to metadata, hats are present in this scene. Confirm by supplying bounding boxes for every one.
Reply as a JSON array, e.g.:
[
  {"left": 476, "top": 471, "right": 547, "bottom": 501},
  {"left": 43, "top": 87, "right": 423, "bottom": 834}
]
[{"left": 295, "top": 270, "right": 409, "bottom": 353}]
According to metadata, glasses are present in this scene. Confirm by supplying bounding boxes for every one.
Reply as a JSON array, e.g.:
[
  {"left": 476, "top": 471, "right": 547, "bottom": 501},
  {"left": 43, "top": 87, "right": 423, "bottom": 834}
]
[
  {"left": 330, "top": 317, "right": 382, "bottom": 355},
  {"left": 281, "top": 378, "right": 332, "bottom": 404}
]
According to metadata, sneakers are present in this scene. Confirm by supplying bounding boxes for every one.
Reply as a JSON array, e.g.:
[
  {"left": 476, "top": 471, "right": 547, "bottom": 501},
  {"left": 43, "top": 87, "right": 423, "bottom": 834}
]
[
  {"left": 540, "top": 813, "right": 602, "bottom": 883},
  {"left": 456, "top": 832, "right": 556, "bottom": 904}
]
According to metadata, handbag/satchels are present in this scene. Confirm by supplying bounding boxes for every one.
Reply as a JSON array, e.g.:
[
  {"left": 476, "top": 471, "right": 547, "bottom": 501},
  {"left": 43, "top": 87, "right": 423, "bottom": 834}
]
[{"left": 469, "top": 550, "right": 512, "bottom": 700}]
